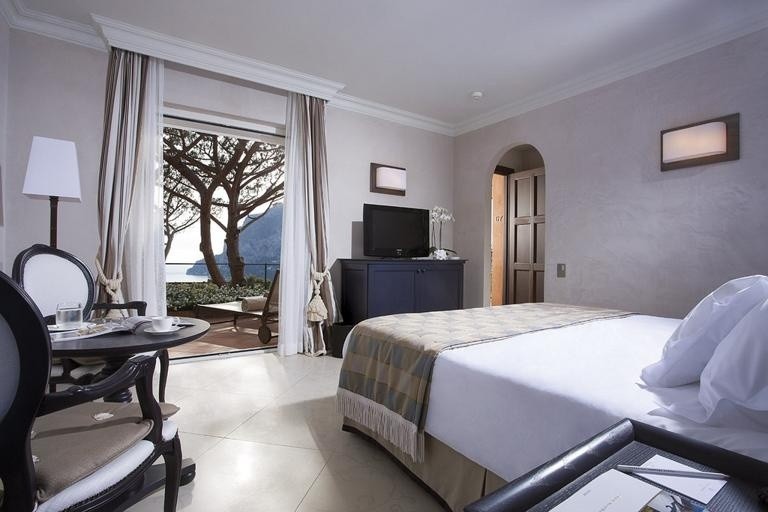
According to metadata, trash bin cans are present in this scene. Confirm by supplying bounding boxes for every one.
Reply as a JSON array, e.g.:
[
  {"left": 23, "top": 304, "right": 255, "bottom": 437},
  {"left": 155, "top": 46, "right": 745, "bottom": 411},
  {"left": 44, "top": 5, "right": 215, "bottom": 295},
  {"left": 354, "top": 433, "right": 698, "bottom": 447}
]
[{"left": 330, "top": 322, "right": 354, "bottom": 358}]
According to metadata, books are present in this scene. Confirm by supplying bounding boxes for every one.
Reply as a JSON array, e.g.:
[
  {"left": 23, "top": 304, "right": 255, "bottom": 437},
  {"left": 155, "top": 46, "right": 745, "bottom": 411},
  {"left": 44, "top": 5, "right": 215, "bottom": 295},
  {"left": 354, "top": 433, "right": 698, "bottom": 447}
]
[
  {"left": 49, "top": 315, "right": 196, "bottom": 343},
  {"left": 548, "top": 470, "right": 713, "bottom": 512}
]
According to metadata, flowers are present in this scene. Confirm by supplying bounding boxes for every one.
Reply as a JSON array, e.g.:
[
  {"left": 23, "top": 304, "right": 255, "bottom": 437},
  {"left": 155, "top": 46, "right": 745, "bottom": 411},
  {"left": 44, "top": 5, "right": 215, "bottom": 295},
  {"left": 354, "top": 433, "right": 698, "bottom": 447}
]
[{"left": 431, "top": 207, "right": 455, "bottom": 249}]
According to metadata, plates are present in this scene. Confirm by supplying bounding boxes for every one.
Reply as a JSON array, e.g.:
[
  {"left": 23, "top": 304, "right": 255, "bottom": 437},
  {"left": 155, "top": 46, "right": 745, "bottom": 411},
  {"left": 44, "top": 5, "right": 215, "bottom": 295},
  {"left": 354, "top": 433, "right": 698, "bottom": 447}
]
[{"left": 141, "top": 326, "right": 188, "bottom": 335}]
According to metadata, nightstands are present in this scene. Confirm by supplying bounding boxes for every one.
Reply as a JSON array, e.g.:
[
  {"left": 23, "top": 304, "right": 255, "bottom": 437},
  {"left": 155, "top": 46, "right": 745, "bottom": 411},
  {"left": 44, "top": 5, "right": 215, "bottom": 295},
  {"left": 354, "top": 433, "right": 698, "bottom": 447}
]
[{"left": 466, "top": 419, "right": 763, "bottom": 510}]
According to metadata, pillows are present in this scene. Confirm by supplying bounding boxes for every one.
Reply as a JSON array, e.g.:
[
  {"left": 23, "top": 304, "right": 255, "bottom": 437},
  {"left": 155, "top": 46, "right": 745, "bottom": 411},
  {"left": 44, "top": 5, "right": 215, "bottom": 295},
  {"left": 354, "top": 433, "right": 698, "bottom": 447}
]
[
  {"left": 640, "top": 273, "right": 768, "bottom": 391},
  {"left": 700, "top": 294, "right": 767, "bottom": 418},
  {"left": 240, "top": 296, "right": 268, "bottom": 311}
]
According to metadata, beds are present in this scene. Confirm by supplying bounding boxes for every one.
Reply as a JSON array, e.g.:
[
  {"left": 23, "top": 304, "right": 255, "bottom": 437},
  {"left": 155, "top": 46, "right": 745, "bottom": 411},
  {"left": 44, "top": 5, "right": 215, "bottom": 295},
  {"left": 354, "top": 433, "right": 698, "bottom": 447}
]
[{"left": 335, "top": 302, "right": 768, "bottom": 509}]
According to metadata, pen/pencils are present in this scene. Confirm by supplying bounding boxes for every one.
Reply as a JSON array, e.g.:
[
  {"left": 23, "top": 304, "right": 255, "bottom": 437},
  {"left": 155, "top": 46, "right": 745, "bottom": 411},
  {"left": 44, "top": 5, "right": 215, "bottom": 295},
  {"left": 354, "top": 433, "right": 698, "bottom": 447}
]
[{"left": 617, "top": 464, "right": 732, "bottom": 479}]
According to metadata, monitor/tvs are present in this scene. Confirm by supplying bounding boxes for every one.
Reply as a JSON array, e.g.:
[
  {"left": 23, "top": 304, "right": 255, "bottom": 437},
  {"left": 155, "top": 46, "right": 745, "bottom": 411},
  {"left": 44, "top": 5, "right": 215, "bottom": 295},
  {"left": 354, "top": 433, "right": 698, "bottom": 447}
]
[{"left": 363, "top": 203, "right": 429, "bottom": 258}]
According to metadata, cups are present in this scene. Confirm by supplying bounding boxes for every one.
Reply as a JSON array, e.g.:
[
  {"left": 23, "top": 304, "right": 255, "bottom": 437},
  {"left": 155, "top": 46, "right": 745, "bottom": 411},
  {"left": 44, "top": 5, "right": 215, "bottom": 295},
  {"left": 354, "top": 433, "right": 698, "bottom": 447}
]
[
  {"left": 151, "top": 315, "right": 178, "bottom": 331},
  {"left": 53, "top": 301, "right": 84, "bottom": 331}
]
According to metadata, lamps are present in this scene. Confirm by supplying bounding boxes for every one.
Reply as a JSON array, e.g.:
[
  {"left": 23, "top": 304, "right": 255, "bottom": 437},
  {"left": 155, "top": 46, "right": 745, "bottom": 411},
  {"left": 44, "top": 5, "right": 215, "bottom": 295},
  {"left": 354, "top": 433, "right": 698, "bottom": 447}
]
[
  {"left": 659, "top": 112, "right": 741, "bottom": 171},
  {"left": 21, "top": 135, "right": 84, "bottom": 247},
  {"left": 369, "top": 162, "right": 407, "bottom": 197}
]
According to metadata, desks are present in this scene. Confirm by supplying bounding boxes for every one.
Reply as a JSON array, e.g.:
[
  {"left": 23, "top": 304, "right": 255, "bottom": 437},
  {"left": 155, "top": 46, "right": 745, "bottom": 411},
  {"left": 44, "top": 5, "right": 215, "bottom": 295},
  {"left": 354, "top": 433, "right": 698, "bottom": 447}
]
[{"left": 32, "top": 316, "right": 209, "bottom": 512}]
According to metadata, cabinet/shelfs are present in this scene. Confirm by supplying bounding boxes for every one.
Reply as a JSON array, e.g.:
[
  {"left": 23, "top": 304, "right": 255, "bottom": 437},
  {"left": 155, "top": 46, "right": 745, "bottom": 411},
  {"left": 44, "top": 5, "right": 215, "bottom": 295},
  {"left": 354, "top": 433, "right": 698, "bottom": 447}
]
[{"left": 340, "top": 258, "right": 464, "bottom": 320}]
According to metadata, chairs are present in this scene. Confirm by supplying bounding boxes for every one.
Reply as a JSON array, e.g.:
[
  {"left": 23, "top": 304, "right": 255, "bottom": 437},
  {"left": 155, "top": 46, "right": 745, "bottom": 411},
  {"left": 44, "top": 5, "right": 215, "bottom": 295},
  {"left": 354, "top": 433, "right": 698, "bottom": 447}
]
[
  {"left": 0, "top": 268, "right": 183, "bottom": 511},
  {"left": 191, "top": 269, "right": 283, "bottom": 343},
  {"left": 11, "top": 244, "right": 171, "bottom": 418}
]
